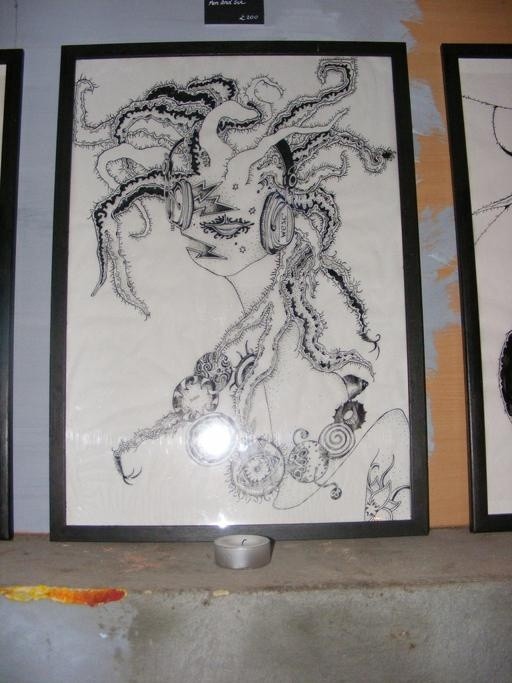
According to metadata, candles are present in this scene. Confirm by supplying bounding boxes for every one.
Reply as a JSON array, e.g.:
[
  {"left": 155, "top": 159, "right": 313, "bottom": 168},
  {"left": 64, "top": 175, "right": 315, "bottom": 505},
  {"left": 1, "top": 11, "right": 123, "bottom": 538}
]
[{"left": 215, "top": 535, "right": 270, "bottom": 570}]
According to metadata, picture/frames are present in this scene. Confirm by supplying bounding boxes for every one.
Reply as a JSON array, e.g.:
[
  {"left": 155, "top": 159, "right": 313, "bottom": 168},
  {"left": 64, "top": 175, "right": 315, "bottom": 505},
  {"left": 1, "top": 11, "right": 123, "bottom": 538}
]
[
  {"left": 1, "top": 48, "right": 25, "bottom": 540},
  {"left": 439, "top": 42, "right": 512, "bottom": 532},
  {"left": 50, "top": 40, "right": 430, "bottom": 543}
]
